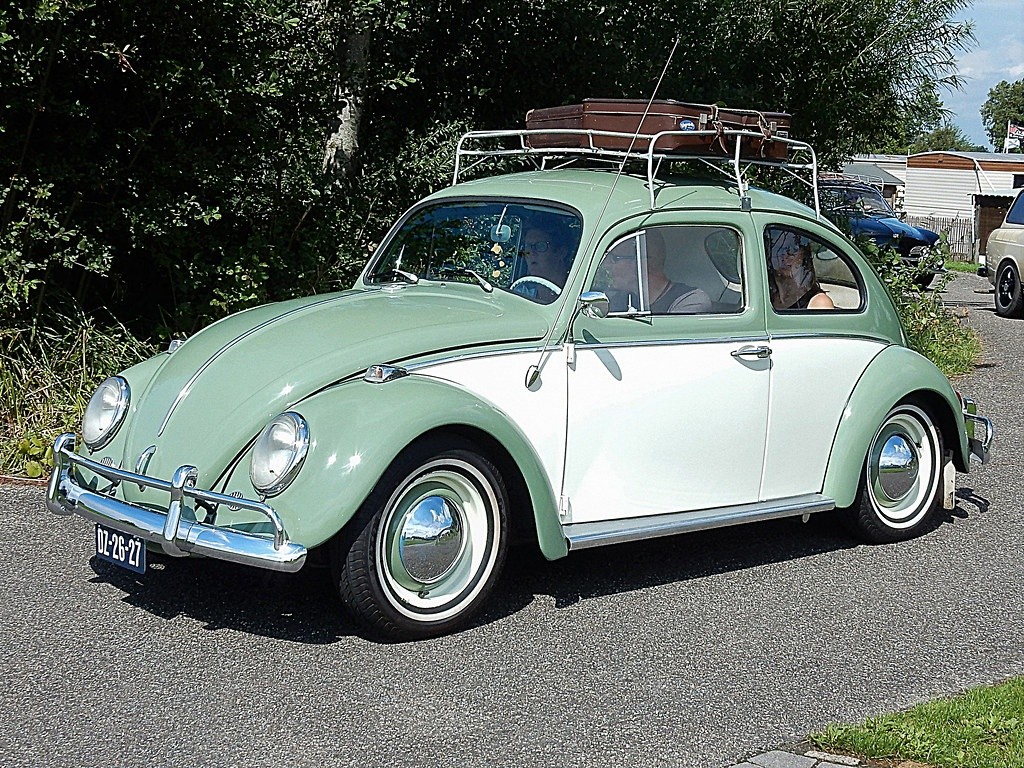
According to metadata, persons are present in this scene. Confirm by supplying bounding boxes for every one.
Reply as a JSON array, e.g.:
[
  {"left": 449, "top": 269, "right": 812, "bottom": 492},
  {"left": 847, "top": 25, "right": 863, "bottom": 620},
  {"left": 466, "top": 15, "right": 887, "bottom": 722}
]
[
  {"left": 846, "top": 190, "right": 860, "bottom": 208},
  {"left": 511, "top": 222, "right": 576, "bottom": 303},
  {"left": 605, "top": 228, "right": 710, "bottom": 315},
  {"left": 766, "top": 236, "right": 835, "bottom": 308}
]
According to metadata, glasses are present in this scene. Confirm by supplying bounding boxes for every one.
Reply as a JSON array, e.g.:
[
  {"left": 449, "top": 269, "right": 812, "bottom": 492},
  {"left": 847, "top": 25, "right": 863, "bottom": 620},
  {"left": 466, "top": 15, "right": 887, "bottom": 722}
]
[
  {"left": 769, "top": 244, "right": 803, "bottom": 257},
  {"left": 607, "top": 252, "right": 653, "bottom": 265},
  {"left": 521, "top": 241, "right": 559, "bottom": 254}
]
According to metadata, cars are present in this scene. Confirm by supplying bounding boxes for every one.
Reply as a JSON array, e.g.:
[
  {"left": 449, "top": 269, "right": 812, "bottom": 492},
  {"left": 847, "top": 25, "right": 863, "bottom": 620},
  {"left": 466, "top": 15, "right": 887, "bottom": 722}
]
[{"left": 986, "top": 190, "right": 1024, "bottom": 314}]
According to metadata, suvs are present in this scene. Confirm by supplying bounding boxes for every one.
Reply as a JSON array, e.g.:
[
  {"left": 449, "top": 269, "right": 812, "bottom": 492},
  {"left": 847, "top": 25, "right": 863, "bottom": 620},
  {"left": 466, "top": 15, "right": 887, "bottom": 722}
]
[
  {"left": 47, "top": 97, "right": 995, "bottom": 645},
  {"left": 776, "top": 170, "right": 942, "bottom": 289}
]
